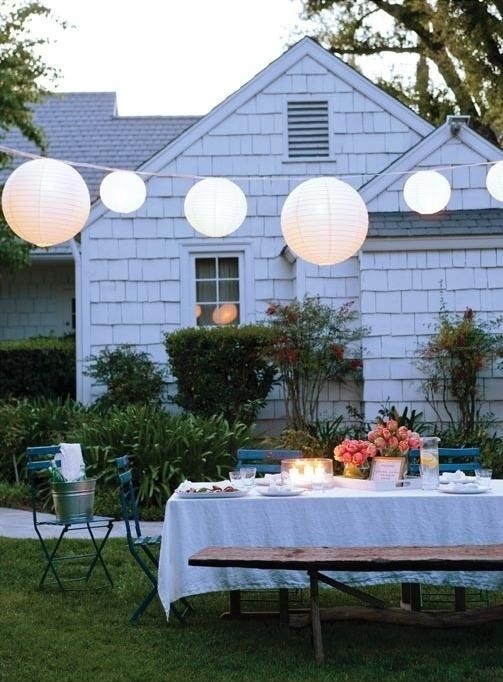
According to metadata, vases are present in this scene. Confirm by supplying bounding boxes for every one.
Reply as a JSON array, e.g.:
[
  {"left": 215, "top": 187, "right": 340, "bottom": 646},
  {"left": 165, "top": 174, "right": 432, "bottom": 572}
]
[{"left": 343, "top": 463, "right": 365, "bottom": 479}]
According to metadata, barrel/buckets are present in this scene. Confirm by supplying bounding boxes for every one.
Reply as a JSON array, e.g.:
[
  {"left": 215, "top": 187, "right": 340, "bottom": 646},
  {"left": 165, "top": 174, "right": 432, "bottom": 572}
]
[{"left": 49, "top": 478, "right": 98, "bottom": 522}]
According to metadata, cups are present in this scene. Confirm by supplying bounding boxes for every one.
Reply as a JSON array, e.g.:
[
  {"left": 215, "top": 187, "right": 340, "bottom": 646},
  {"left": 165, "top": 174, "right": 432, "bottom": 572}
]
[
  {"left": 475, "top": 468, "right": 492, "bottom": 484},
  {"left": 419, "top": 437, "right": 440, "bottom": 489},
  {"left": 228, "top": 472, "right": 245, "bottom": 486},
  {"left": 241, "top": 467, "right": 256, "bottom": 483}
]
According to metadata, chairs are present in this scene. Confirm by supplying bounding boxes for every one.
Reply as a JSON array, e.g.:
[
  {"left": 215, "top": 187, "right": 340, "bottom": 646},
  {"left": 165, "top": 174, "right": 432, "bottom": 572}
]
[
  {"left": 235, "top": 449, "right": 305, "bottom": 478},
  {"left": 409, "top": 449, "right": 482, "bottom": 479},
  {"left": 30, "top": 445, "right": 118, "bottom": 598},
  {"left": 115, "top": 454, "right": 194, "bottom": 625}
]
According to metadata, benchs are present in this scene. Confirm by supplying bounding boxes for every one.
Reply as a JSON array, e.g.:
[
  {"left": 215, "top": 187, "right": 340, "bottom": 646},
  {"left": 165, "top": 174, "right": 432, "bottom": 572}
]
[{"left": 187, "top": 545, "right": 503, "bottom": 664}]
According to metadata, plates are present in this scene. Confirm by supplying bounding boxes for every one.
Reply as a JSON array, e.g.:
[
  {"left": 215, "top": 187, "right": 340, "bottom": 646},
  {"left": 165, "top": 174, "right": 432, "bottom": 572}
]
[
  {"left": 336, "top": 474, "right": 419, "bottom": 492},
  {"left": 178, "top": 490, "right": 250, "bottom": 498},
  {"left": 255, "top": 478, "right": 278, "bottom": 486},
  {"left": 441, "top": 476, "right": 475, "bottom": 483},
  {"left": 254, "top": 487, "right": 306, "bottom": 496},
  {"left": 440, "top": 485, "right": 493, "bottom": 494}
]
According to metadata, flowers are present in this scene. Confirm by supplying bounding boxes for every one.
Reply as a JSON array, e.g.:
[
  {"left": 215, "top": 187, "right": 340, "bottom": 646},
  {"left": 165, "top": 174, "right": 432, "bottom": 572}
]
[
  {"left": 366, "top": 417, "right": 421, "bottom": 457},
  {"left": 334, "top": 438, "right": 376, "bottom": 464}
]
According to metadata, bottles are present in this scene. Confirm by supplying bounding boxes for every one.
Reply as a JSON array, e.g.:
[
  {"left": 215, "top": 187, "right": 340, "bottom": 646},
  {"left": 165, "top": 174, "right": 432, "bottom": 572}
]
[
  {"left": 77, "top": 463, "right": 87, "bottom": 482},
  {"left": 47, "top": 466, "right": 68, "bottom": 482},
  {"left": 51, "top": 456, "right": 66, "bottom": 482}
]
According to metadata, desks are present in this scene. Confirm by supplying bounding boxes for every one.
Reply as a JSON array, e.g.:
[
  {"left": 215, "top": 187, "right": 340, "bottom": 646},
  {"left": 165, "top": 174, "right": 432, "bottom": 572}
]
[{"left": 167, "top": 475, "right": 503, "bottom": 625}]
